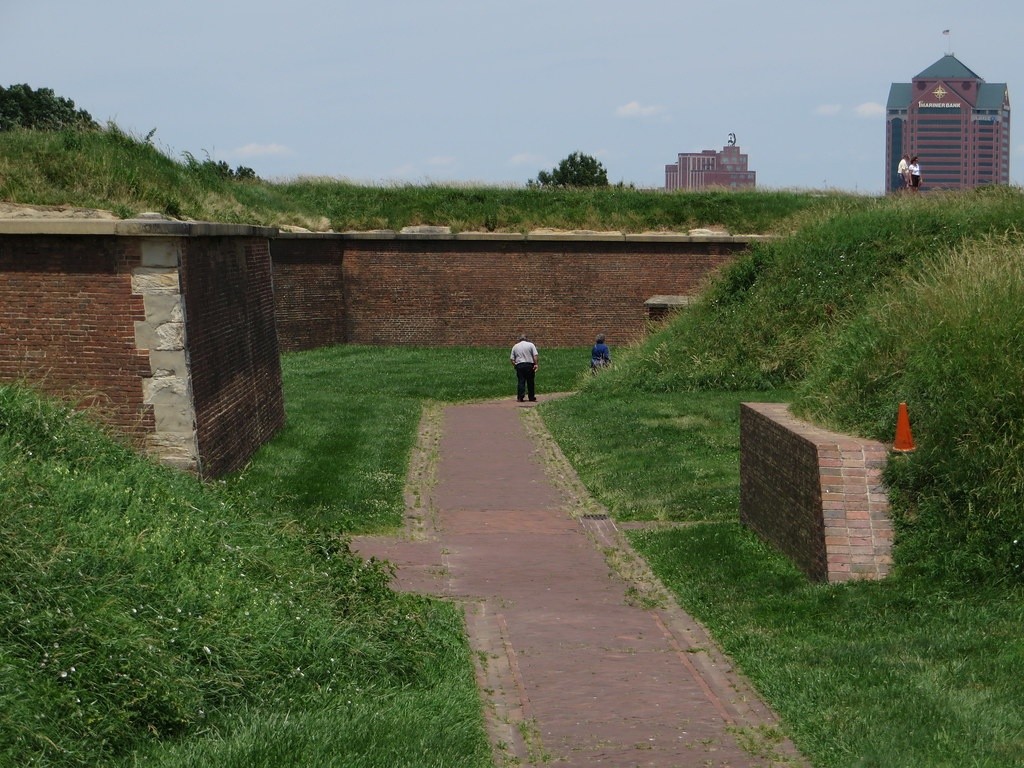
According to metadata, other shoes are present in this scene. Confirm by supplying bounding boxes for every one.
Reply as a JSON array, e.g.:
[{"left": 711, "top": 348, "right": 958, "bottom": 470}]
[
  {"left": 517, "top": 398, "right": 523, "bottom": 402},
  {"left": 529, "top": 398, "right": 536, "bottom": 401}
]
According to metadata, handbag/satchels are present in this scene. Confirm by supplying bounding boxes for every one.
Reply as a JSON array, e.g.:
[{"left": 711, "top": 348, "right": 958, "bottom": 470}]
[{"left": 591, "top": 357, "right": 603, "bottom": 368}]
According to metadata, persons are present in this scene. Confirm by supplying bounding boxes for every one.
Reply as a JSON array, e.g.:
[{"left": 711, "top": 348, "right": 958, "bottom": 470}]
[
  {"left": 591, "top": 333, "right": 611, "bottom": 373},
  {"left": 897, "top": 153, "right": 911, "bottom": 190},
  {"left": 510, "top": 333, "right": 539, "bottom": 402},
  {"left": 908, "top": 156, "right": 923, "bottom": 194}
]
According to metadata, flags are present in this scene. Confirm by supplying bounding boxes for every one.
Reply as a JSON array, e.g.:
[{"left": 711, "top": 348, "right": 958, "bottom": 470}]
[{"left": 943, "top": 30, "right": 949, "bottom": 35}]
